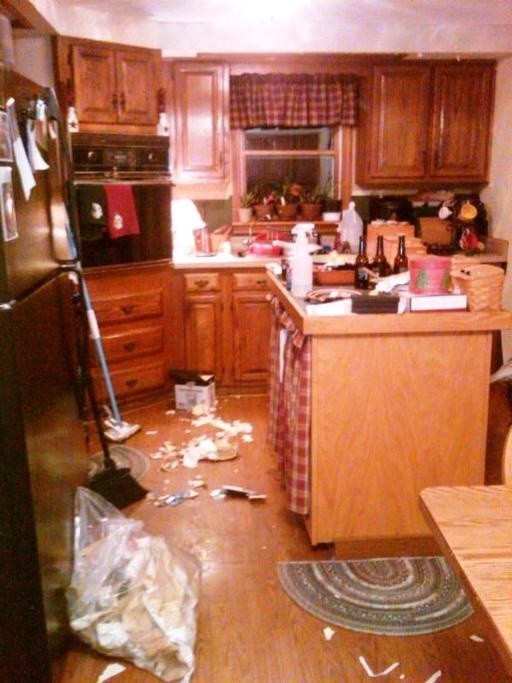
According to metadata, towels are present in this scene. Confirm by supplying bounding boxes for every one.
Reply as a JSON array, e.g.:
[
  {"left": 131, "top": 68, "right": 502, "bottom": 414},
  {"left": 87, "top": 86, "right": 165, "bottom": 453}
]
[{"left": 103, "top": 182, "right": 142, "bottom": 240}]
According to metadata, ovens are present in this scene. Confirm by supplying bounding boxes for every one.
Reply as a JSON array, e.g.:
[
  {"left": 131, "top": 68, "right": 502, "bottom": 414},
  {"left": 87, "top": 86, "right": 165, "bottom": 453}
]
[{"left": 67, "top": 127, "right": 173, "bottom": 270}]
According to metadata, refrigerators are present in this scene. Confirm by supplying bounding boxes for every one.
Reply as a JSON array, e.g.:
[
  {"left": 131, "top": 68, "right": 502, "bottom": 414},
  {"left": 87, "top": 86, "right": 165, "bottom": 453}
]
[{"left": 2, "top": 68, "right": 98, "bottom": 673}]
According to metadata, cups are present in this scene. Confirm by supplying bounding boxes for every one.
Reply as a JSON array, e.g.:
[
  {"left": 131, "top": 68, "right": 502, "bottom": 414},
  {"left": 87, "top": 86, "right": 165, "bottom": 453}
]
[
  {"left": 459, "top": 200, "right": 478, "bottom": 222},
  {"left": 410, "top": 257, "right": 452, "bottom": 297}
]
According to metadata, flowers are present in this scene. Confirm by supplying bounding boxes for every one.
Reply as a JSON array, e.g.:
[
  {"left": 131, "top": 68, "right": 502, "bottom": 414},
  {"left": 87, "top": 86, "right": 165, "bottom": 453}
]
[{"left": 233, "top": 170, "right": 335, "bottom": 222}]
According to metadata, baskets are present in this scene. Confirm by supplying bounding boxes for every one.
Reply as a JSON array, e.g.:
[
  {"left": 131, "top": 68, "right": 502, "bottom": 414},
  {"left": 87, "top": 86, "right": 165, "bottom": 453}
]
[
  {"left": 418, "top": 216, "right": 454, "bottom": 248},
  {"left": 448, "top": 263, "right": 505, "bottom": 312}
]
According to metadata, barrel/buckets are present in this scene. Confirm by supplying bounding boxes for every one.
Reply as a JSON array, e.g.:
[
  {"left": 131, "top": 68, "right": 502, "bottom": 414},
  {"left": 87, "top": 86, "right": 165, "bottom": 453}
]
[{"left": 340, "top": 200, "right": 363, "bottom": 254}]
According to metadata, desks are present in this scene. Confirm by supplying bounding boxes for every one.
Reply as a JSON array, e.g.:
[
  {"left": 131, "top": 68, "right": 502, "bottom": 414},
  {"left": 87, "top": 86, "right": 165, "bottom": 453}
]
[
  {"left": 264, "top": 265, "right": 512, "bottom": 550},
  {"left": 416, "top": 483, "right": 511, "bottom": 677}
]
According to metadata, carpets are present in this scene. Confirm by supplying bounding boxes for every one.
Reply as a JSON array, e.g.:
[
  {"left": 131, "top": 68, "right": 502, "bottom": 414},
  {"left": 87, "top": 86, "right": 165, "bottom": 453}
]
[{"left": 275, "top": 554, "right": 477, "bottom": 638}]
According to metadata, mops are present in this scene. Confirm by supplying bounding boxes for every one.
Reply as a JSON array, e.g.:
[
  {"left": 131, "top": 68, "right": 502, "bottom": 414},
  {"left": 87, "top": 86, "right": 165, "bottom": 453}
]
[{"left": 63, "top": 222, "right": 142, "bottom": 443}]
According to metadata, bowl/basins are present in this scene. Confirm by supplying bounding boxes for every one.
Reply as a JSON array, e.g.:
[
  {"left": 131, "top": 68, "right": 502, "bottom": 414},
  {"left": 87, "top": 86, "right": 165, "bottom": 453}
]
[{"left": 314, "top": 268, "right": 356, "bottom": 287}]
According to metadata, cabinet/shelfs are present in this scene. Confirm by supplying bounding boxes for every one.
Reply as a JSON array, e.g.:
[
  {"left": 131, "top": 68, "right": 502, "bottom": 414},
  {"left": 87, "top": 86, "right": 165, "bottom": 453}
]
[
  {"left": 355, "top": 57, "right": 492, "bottom": 189},
  {"left": 70, "top": 39, "right": 160, "bottom": 124},
  {"left": 180, "top": 269, "right": 226, "bottom": 384},
  {"left": 85, "top": 269, "right": 177, "bottom": 401},
  {"left": 172, "top": 58, "right": 230, "bottom": 183},
  {"left": 227, "top": 269, "right": 274, "bottom": 384}
]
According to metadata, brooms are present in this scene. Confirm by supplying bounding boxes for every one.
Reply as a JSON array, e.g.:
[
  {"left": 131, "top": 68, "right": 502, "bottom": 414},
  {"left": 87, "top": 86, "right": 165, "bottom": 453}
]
[{"left": 74, "top": 343, "right": 149, "bottom": 510}]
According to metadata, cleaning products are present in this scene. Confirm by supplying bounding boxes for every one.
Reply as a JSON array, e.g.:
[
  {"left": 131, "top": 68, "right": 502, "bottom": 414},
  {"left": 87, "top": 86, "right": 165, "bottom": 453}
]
[{"left": 290, "top": 222, "right": 315, "bottom": 298}]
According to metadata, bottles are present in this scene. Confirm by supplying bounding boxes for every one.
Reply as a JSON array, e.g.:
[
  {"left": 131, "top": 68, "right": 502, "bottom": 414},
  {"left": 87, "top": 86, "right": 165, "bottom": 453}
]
[
  {"left": 355, "top": 232, "right": 409, "bottom": 288},
  {"left": 339, "top": 202, "right": 365, "bottom": 251}
]
